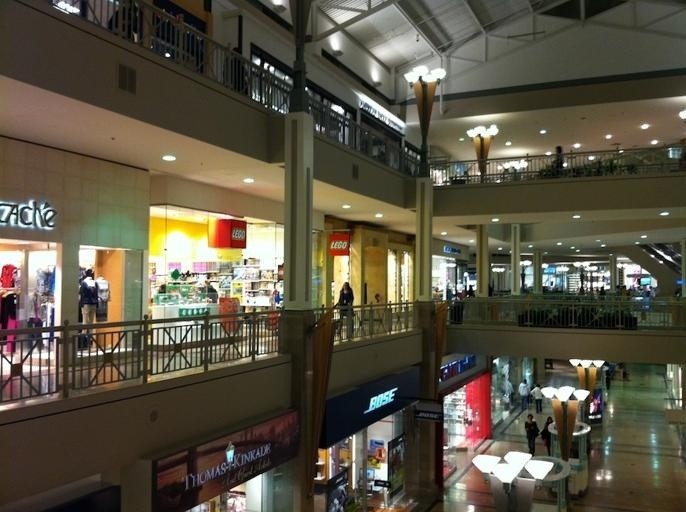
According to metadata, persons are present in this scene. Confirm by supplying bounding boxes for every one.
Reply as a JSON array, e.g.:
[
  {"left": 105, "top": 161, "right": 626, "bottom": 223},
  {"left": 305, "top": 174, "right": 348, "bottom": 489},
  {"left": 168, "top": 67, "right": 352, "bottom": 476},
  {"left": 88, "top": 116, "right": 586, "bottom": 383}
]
[
  {"left": 540, "top": 416, "right": 553, "bottom": 456},
  {"left": 375, "top": 294, "right": 383, "bottom": 304},
  {"left": 202, "top": 280, "right": 218, "bottom": 303},
  {"left": 223, "top": 47, "right": 250, "bottom": 95},
  {"left": 466, "top": 422, "right": 474, "bottom": 454},
  {"left": 518, "top": 379, "right": 530, "bottom": 413},
  {"left": 335, "top": 282, "right": 353, "bottom": 334},
  {"left": 530, "top": 384, "right": 543, "bottom": 413},
  {"left": 499, "top": 376, "right": 513, "bottom": 410},
  {"left": 615, "top": 358, "right": 630, "bottom": 380},
  {"left": 577, "top": 283, "right": 655, "bottom": 303},
  {"left": 525, "top": 415, "right": 539, "bottom": 457},
  {"left": 552, "top": 147, "right": 564, "bottom": 178},
  {"left": 431, "top": 282, "right": 474, "bottom": 303},
  {"left": 78, "top": 275, "right": 97, "bottom": 351},
  {"left": 95, "top": 276, "right": 110, "bottom": 323}
]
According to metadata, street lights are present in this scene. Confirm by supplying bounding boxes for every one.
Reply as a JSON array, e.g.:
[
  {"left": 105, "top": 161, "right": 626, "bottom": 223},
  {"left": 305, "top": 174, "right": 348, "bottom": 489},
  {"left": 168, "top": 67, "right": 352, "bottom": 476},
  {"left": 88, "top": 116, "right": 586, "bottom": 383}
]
[
  {"left": 541, "top": 386, "right": 590, "bottom": 503},
  {"left": 405, "top": 65, "right": 447, "bottom": 177},
  {"left": 467, "top": 124, "right": 499, "bottom": 183},
  {"left": 570, "top": 359, "right": 605, "bottom": 454},
  {"left": 472, "top": 451, "right": 555, "bottom": 512}
]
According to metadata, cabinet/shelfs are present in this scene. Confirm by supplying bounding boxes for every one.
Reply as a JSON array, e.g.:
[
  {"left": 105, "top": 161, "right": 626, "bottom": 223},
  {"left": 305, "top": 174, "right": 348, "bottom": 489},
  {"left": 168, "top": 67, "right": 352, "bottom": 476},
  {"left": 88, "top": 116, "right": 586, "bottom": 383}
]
[{"left": 191, "top": 263, "right": 277, "bottom": 294}]
